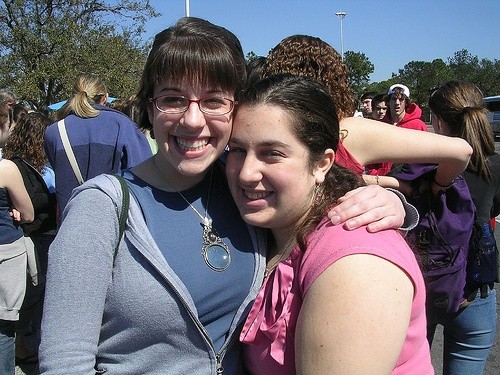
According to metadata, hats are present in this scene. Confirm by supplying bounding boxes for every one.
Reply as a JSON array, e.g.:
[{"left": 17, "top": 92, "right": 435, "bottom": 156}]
[{"left": 386, "top": 83, "right": 411, "bottom": 100}]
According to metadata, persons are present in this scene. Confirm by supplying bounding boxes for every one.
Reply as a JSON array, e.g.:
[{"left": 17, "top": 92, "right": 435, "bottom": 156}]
[
  {"left": 108, "top": 95, "right": 158, "bottom": 157},
  {"left": 44, "top": 75, "right": 154, "bottom": 234},
  {"left": 0, "top": 93, "right": 35, "bottom": 375},
  {"left": 0, "top": 93, "right": 28, "bottom": 134},
  {"left": 348, "top": 83, "right": 427, "bottom": 177},
  {"left": 385, "top": 79, "right": 500, "bottom": 375},
  {"left": 2, "top": 112, "right": 56, "bottom": 362},
  {"left": 38, "top": 16, "right": 420, "bottom": 375},
  {"left": 226, "top": 73, "right": 435, "bottom": 375},
  {"left": 260, "top": 34, "right": 473, "bottom": 200}
]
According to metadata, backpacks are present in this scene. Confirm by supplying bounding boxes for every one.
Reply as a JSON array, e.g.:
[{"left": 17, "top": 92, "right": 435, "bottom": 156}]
[{"left": 383, "top": 162, "right": 476, "bottom": 315}]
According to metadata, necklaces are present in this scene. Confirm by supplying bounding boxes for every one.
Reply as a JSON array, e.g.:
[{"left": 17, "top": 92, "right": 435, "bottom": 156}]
[{"left": 153, "top": 155, "right": 232, "bottom": 272}]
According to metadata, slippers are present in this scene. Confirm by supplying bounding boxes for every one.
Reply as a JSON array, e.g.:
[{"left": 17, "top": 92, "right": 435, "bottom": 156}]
[{"left": 15, "top": 351, "right": 39, "bottom": 365}]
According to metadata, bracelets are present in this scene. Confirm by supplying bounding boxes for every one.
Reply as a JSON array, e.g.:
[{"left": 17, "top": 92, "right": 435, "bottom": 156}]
[
  {"left": 375, "top": 174, "right": 379, "bottom": 185},
  {"left": 434, "top": 179, "right": 455, "bottom": 188}
]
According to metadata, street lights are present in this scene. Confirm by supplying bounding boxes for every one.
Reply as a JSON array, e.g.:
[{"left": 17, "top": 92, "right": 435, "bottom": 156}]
[{"left": 334, "top": 11, "right": 347, "bottom": 62}]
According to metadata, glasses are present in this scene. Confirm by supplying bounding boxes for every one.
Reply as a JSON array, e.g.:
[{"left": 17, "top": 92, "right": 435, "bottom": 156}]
[{"left": 149, "top": 95, "right": 239, "bottom": 117}]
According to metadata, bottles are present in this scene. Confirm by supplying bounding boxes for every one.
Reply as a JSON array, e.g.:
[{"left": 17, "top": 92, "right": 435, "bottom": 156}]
[{"left": 479, "top": 223, "right": 497, "bottom": 284}]
